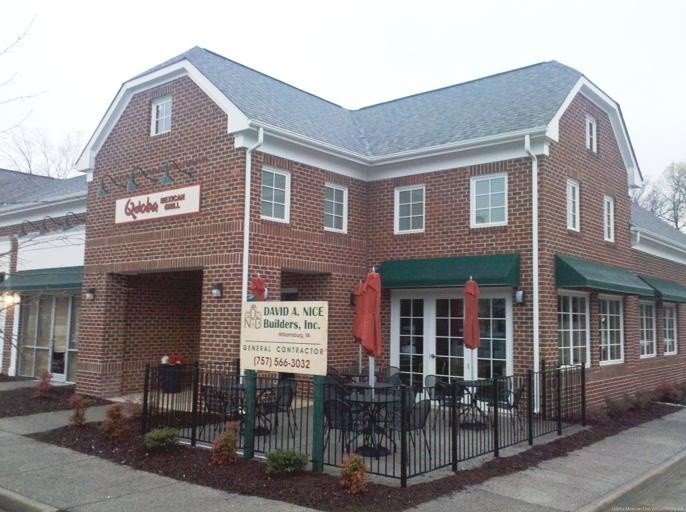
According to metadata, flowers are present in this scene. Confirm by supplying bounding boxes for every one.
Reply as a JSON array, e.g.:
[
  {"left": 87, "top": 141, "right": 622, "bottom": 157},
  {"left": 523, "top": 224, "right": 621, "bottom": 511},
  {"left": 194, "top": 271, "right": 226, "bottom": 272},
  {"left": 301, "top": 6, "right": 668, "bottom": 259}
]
[{"left": 160, "top": 354, "right": 186, "bottom": 366}]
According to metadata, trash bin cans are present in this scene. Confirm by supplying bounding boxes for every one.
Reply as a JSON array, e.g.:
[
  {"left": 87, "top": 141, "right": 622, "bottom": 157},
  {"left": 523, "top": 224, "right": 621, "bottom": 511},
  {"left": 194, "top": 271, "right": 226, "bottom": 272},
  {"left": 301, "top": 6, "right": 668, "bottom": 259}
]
[{"left": 159, "top": 364, "right": 182, "bottom": 393}]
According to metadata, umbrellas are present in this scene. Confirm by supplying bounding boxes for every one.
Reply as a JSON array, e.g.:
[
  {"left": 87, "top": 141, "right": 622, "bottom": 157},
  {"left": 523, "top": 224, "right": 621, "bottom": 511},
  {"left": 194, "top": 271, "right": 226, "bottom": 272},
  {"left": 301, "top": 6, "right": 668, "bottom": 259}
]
[
  {"left": 462, "top": 275, "right": 481, "bottom": 350},
  {"left": 352, "top": 278, "right": 364, "bottom": 344},
  {"left": 359, "top": 271, "right": 381, "bottom": 360},
  {"left": 249, "top": 272, "right": 266, "bottom": 302}
]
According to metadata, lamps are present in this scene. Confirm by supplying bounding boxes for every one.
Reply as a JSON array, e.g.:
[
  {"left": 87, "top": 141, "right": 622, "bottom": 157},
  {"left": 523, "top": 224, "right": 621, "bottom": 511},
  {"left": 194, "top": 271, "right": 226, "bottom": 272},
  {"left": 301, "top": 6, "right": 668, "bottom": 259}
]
[
  {"left": 63, "top": 212, "right": 84, "bottom": 231},
  {"left": 516, "top": 289, "right": 528, "bottom": 304},
  {"left": 18, "top": 220, "right": 39, "bottom": 238},
  {"left": 160, "top": 161, "right": 191, "bottom": 185},
  {"left": 86, "top": 288, "right": 96, "bottom": 300},
  {"left": 126, "top": 168, "right": 159, "bottom": 191},
  {"left": 96, "top": 174, "right": 126, "bottom": 196},
  {"left": 210, "top": 283, "right": 222, "bottom": 298},
  {"left": 40, "top": 216, "right": 63, "bottom": 235}
]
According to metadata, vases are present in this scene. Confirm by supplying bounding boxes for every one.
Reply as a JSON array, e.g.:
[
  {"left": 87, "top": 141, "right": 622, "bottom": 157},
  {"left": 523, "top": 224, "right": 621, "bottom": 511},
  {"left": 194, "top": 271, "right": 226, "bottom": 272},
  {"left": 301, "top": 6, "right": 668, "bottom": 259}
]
[{"left": 157, "top": 364, "right": 185, "bottom": 393}]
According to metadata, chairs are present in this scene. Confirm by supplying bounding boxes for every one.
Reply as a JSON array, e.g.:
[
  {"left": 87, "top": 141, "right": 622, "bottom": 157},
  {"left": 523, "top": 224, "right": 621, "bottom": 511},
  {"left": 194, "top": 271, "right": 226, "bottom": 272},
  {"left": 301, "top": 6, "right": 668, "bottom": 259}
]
[
  {"left": 199, "top": 377, "right": 298, "bottom": 443},
  {"left": 323, "top": 366, "right": 525, "bottom": 459}
]
